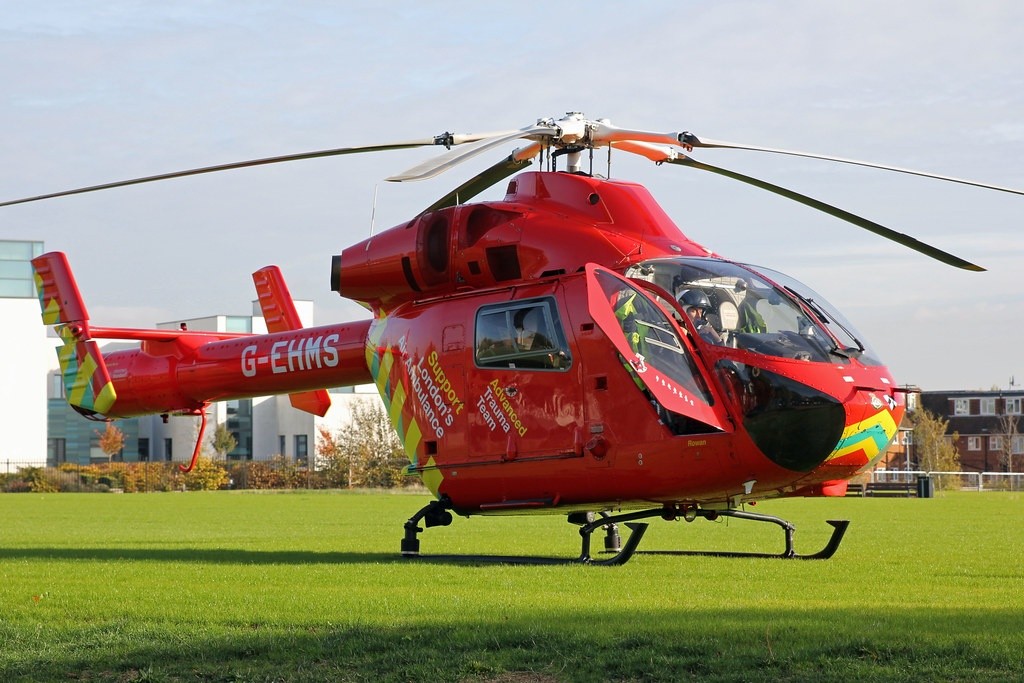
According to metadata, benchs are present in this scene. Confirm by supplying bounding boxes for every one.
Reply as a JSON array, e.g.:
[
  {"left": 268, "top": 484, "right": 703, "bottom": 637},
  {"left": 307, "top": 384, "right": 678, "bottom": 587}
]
[
  {"left": 846, "top": 483, "right": 864, "bottom": 498},
  {"left": 866, "top": 482, "right": 917, "bottom": 496}
]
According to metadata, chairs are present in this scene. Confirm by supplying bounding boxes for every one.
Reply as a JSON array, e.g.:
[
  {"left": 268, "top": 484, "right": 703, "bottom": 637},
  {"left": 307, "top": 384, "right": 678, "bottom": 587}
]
[{"left": 719, "top": 302, "right": 740, "bottom": 347}]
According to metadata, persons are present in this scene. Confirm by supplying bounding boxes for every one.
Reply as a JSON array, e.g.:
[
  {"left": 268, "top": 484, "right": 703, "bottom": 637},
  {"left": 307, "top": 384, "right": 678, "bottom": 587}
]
[
  {"left": 672, "top": 288, "right": 753, "bottom": 394},
  {"left": 613, "top": 265, "right": 686, "bottom": 430}
]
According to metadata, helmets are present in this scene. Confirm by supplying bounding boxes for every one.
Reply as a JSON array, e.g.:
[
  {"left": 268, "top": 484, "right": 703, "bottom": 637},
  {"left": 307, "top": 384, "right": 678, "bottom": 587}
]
[{"left": 679, "top": 290, "right": 713, "bottom": 311}]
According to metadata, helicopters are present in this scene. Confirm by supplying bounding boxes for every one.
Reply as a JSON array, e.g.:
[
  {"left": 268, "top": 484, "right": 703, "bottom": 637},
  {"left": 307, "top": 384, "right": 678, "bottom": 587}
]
[{"left": 0, "top": 109, "right": 1024, "bottom": 565}]
